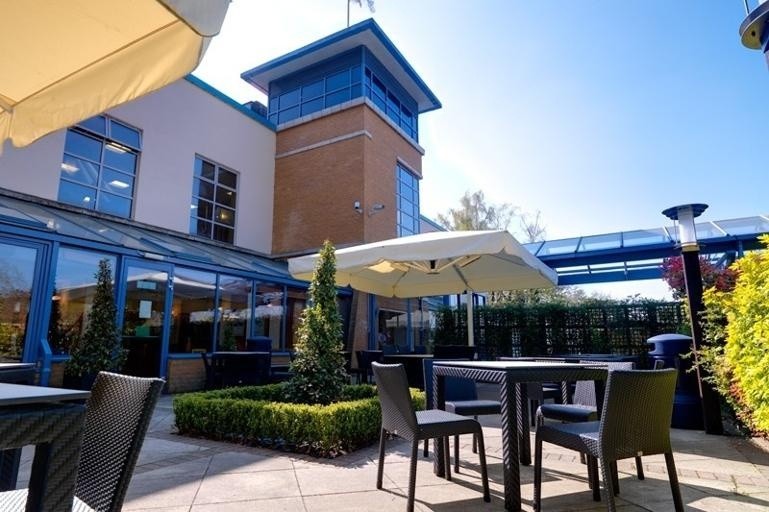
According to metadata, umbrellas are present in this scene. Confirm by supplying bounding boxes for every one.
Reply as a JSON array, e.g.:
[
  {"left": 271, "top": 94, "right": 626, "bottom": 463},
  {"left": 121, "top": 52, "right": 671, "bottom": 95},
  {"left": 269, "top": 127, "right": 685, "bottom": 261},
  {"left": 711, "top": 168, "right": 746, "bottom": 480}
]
[
  {"left": 287, "top": 224, "right": 561, "bottom": 360},
  {"left": 0, "top": 0, "right": 248, "bottom": 155}
]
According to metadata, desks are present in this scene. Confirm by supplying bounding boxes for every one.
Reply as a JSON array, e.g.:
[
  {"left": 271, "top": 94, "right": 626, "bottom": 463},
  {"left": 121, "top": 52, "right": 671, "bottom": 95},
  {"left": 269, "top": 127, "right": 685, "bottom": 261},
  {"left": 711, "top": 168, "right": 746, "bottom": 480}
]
[
  {"left": 429, "top": 360, "right": 637, "bottom": 511},
  {"left": 0, "top": 360, "right": 93, "bottom": 511}
]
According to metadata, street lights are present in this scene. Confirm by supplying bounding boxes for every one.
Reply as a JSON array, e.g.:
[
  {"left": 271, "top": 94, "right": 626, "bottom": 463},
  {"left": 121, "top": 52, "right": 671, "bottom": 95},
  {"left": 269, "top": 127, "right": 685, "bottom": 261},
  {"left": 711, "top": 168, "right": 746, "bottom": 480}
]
[{"left": 662, "top": 199, "right": 721, "bottom": 433}]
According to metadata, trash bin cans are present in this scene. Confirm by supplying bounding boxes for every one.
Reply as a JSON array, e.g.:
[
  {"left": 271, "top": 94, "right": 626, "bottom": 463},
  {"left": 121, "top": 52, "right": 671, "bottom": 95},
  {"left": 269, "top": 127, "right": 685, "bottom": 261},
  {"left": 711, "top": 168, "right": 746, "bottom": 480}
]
[
  {"left": 247, "top": 336, "right": 272, "bottom": 378},
  {"left": 646, "top": 333, "right": 697, "bottom": 429}
]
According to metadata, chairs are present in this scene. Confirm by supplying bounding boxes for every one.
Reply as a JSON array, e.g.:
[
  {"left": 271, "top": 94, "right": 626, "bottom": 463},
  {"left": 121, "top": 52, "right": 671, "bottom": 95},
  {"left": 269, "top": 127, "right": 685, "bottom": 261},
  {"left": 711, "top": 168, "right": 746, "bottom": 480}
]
[
  {"left": 420, "top": 358, "right": 503, "bottom": 472},
  {"left": 0, "top": 370, "right": 166, "bottom": 512},
  {"left": 370, "top": 361, "right": 489, "bottom": 512},
  {"left": 529, "top": 359, "right": 645, "bottom": 479},
  {"left": 531, "top": 367, "right": 683, "bottom": 511}
]
